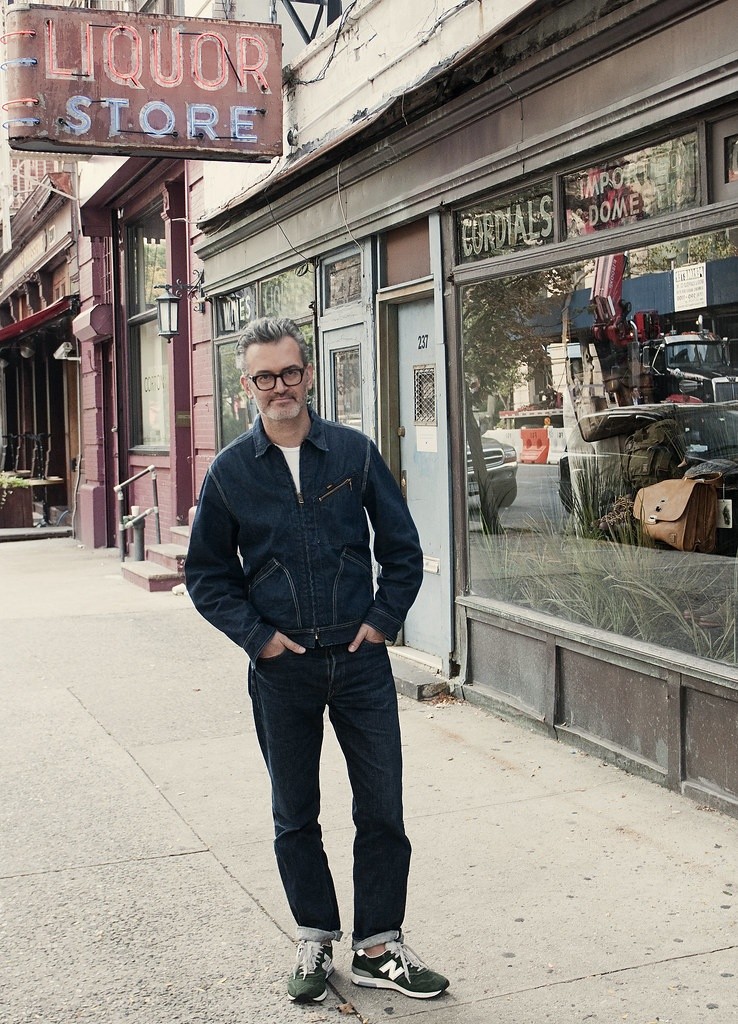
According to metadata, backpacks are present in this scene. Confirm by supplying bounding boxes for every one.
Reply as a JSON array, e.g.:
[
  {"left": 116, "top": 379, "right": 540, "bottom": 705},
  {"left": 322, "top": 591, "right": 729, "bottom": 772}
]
[{"left": 622, "top": 419, "right": 688, "bottom": 490}]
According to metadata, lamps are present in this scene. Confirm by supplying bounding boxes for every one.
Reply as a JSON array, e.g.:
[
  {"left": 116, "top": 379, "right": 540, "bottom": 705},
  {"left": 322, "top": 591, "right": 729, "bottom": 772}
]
[
  {"left": 20, "top": 337, "right": 37, "bottom": 358},
  {"left": 153, "top": 268, "right": 205, "bottom": 344}
]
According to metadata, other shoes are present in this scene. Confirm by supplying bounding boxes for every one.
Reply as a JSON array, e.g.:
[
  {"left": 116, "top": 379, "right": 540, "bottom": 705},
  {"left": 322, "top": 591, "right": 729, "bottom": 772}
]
[
  {"left": 684, "top": 588, "right": 730, "bottom": 620},
  {"left": 698, "top": 593, "right": 734, "bottom": 627},
  {"left": 589, "top": 494, "right": 635, "bottom": 532}
]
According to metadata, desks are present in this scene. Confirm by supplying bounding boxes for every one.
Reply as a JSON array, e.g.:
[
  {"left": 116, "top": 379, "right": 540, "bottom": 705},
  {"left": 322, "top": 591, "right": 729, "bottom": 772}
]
[{"left": 23, "top": 478, "right": 64, "bottom": 527}]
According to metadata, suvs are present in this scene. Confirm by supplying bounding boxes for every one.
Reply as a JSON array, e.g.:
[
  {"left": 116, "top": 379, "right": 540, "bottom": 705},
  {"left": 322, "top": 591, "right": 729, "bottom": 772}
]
[
  {"left": 466, "top": 435, "right": 518, "bottom": 508},
  {"left": 558, "top": 402, "right": 738, "bottom": 554}
]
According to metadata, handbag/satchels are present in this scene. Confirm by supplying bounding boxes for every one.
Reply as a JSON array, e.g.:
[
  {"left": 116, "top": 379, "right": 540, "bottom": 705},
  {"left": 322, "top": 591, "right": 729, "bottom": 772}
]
[{"left": 631, "top": 469, "right": 723, "bottom": 555}]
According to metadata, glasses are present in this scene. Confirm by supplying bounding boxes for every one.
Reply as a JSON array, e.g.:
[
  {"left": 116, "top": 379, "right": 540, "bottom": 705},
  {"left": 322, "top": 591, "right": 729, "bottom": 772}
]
[{"left": 247, "top": 361, "right": 308, "bottom": 392}]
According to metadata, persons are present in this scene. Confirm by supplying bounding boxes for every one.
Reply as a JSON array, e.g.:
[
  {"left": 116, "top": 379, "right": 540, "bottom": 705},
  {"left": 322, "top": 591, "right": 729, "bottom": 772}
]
[{"left": 185, "top": 317, "right": 449, "bottom": 1003}]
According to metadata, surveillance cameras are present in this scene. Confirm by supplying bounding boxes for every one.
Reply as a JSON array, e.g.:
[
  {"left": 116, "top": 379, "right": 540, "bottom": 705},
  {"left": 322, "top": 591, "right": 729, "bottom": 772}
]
[{"left": 53, "top": 342, "right": 71, "bottom": 360}]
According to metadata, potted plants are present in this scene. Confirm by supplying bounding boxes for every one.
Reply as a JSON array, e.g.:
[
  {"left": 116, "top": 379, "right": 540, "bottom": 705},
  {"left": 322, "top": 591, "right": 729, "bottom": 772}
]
[{"left": 0, "top": 471, "right": 33, "bottom": 529}]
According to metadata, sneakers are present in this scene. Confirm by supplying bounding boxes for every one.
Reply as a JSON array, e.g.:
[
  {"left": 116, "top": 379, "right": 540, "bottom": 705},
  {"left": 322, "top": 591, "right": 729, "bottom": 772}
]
[
  {"left": 286, "top": 939, "right": 336, "bottom": 1002},
  {"left": 350, "top": 940, "right": 450, "bottom": 999}
]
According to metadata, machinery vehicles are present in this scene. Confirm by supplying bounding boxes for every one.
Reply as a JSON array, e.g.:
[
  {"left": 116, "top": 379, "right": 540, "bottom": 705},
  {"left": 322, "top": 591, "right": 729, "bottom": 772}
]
[{"left": 559, "top": 252, "right": 738, "bottom": 407}]
